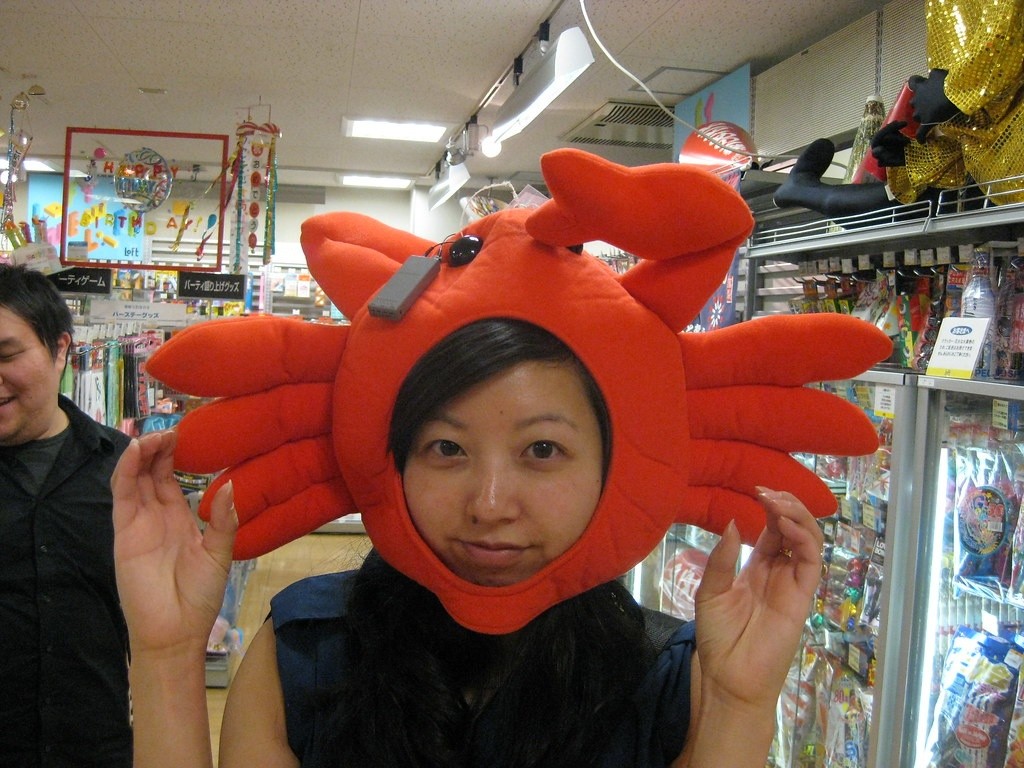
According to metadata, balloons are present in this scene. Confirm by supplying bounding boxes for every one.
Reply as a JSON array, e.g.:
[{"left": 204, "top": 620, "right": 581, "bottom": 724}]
[{"left": 112, "top": 148, "right": 172, "bottom": 216}]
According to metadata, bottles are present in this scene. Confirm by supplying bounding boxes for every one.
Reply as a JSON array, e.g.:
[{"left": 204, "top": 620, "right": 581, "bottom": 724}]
[{"left": 960, "top": 246, "right": 997, "bottom": 381}]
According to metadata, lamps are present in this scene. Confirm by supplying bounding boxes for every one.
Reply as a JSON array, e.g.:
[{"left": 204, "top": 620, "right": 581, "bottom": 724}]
[
  {"left": 425, "top": 150, "right": 471, "bottom": 212},
  {"left": 492, "top": 24, "right": 595, "bottom": 149},
  {"left": 465, "top": 115, "right": 488, "bottom": 152}
]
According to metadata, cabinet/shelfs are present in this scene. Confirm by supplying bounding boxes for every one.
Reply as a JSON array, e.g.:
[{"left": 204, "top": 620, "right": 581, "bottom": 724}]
[
  {"left": 68, "top": 241, "right": 350, "bottom": 331},
  {"left": 632, "top": 176, "right": 1024, "bottom": 768}
]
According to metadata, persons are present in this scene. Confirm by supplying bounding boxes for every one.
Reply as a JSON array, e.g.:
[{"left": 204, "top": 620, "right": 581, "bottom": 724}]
[
  {"left": 0, "top": 262, "right": 139, "bottom": 768},
  {"left": 108, "top": 144, "right": 894, "bottom": 768},
  {"left": 774, "top": 0, "right": 1023, "bottom": 231}
]
